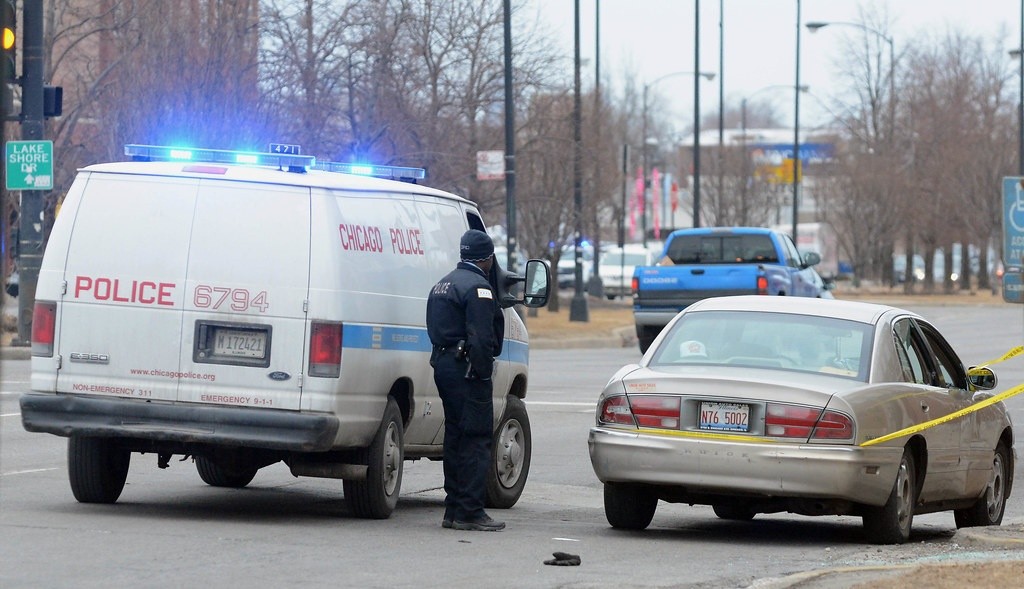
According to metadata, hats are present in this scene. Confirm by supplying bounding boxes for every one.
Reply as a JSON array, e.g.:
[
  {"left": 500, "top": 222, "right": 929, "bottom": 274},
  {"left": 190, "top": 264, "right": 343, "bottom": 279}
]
[{"left": 460, "top": 229, "right": 494, "bottom": 263}]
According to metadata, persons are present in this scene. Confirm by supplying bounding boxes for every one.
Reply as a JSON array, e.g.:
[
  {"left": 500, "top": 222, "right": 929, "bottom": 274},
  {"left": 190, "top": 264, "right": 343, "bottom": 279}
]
[
  {"left": 975, "top": 376, "right": 984, "bottom": 385},
  {"left": 426, "top": 230, "right": 506, "bottom": 531}
]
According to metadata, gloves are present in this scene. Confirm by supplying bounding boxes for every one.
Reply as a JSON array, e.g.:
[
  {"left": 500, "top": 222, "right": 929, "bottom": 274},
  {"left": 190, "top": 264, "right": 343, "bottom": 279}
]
[{"left": 544, "top": 551, "right": 581, "bottom": 565}]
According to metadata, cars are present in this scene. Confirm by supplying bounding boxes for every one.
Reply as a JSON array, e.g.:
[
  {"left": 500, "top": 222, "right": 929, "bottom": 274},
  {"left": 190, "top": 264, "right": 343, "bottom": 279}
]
[
  {"left": 587, "top": 296, "right": 1018, "bottom": 544},
  {"left": 487, "top": 224, "right": 665, "bottom": 300},
  {"left": 774, "top": 224, "right": 1005, "bottom": 285}
]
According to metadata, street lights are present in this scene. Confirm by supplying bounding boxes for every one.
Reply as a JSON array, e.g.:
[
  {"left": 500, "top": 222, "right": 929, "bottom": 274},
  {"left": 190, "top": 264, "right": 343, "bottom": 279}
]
[
  {"left": 741, "top": 84, "right": 809, "bottom": 227},
  {"left": 806, "top": 21, "right": 895, "bottom": 136},
  {"left": 642, "top": 72, "right": 716, "bottom": 249}
]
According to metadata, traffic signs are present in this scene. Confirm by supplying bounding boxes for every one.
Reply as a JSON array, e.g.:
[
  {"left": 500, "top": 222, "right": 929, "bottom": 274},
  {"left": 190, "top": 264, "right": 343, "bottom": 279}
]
[{"left": 5, "top": 140, "right": 55, "bottom": 192}]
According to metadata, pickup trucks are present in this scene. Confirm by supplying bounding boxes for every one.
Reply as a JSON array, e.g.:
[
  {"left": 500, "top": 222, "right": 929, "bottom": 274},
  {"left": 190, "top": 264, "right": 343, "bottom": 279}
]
[{"left": 632, "top": 213, "right": 835, "bottom": 355}]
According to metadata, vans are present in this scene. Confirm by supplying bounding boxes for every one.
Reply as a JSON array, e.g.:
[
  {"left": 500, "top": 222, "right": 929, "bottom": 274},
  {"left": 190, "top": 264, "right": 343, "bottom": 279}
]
[{"left": 20, "top": 143, "right": 550, "bottom": 519}]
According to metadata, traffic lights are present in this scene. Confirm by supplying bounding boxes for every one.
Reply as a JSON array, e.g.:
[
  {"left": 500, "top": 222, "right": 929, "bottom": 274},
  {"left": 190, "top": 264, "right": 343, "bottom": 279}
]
[
  {"left": 0, "top": 0, "right": 18, "bottom": 114},
  {"left": 44, "top": 85, "right": 63, "bottom": 117}
]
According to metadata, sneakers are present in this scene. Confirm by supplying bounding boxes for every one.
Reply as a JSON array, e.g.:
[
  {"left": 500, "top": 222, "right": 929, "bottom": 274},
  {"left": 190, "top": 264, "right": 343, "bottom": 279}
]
[
  {"left": 442, "top": 512, "right": 453, "bottom": 528},
  {"left": 452, "top": 513, "right": 506, "bottom": 530}
]
